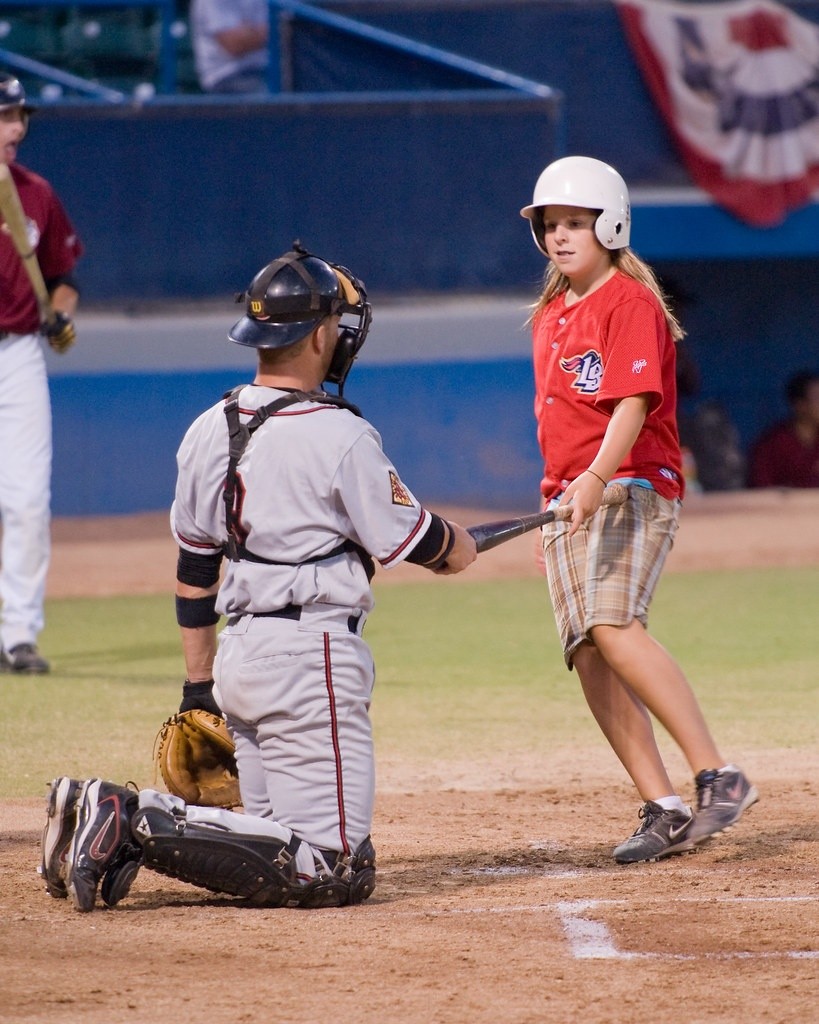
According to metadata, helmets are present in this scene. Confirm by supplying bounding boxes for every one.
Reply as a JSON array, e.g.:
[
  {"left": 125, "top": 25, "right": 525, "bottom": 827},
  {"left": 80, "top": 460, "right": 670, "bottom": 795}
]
[
  {"left": 520, "top": 156, "right": 631, "bottom": 259},
  {"left": 0, "top": 70, "right": 38, "bottom": 114},
  {"left": 225, "top": 252, "right": 345, "bottom": 348}
]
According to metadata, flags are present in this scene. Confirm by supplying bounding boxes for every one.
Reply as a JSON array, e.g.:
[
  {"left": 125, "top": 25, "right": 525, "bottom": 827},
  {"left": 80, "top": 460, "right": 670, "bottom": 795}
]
[{"left": 617, "top": 1, "right": 818, "bottom": 227}]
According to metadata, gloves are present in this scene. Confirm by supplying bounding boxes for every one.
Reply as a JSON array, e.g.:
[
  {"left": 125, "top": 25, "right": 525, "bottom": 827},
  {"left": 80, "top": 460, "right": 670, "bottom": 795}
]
[{"left": 41, "top": 310, "right": 75, "bottom": 354}]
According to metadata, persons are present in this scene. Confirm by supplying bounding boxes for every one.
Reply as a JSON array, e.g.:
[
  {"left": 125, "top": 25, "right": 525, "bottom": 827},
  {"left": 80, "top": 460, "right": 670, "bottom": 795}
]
[
  {"left": 190, "top": 0, "right": 269, "bottom": 96},
  {"left": 0, "top": 73, "right": 83, "bottom": 674},
  {"left": 39, "top": 241, "right": 478, "bottom": 910},
  {"left": 744, "top": 369, "right": 819, "bottom": 489},
  {"left": 521, "top": 155, "right": 758, "bottom": 864}
]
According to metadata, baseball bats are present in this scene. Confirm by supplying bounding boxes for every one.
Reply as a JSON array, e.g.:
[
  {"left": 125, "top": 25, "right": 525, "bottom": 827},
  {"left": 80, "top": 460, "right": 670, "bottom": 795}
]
[
  {"left": 1, "top": 162, "right": 58, "bottom": 325},
  {"left": 436, "top": 482, "right": 628, "bottom": 573}
]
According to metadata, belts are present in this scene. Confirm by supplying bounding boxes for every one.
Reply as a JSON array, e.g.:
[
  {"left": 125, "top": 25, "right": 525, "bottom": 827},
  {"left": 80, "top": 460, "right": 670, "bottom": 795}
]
[{"left": 255, "top": 604, "right": 363, "bottom": 633}]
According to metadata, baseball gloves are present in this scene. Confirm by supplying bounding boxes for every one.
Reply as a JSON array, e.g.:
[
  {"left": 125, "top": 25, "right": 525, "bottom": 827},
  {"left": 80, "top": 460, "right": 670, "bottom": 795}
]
[{"left": 151, "top": 708, "right": 244, "bottom": 810}]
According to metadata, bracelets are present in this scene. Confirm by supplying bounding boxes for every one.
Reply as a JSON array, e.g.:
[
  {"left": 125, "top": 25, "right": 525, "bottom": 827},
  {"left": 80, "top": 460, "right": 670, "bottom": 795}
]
[{"left": 587, "top": 470, "right": 607, "bottom": 488}]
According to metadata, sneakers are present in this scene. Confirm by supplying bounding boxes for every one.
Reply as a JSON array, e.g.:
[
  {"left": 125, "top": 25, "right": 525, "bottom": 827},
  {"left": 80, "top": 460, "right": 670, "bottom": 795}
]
[
  {"left": 690, "top": 765, "right": 759, "bottom": 844},
  {"left": 38, "top": 779, "right": 85, "bottom": 902},
  {"left": 613, "top": 799, "right": 697, "bottom": 864},
  {"left": 0, "top": 642, "right": 48, "bottom": 675},
  {"left": 68, "top": 778, "right": 140, "bottom": 911}
]
